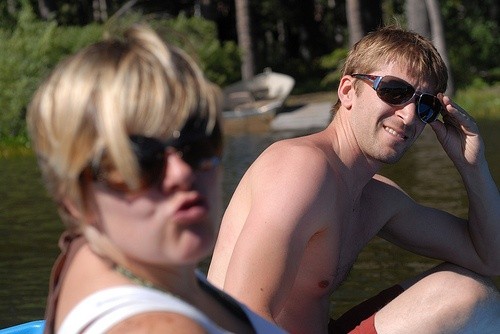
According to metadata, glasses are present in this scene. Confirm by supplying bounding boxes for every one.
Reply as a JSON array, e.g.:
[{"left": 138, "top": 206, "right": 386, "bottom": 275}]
[
  {"left": 78, "top": 104, "right": 225, "bottom": 192},
  {"left": 351, "top": 73, "right": 444, "bottom": 124}
]
